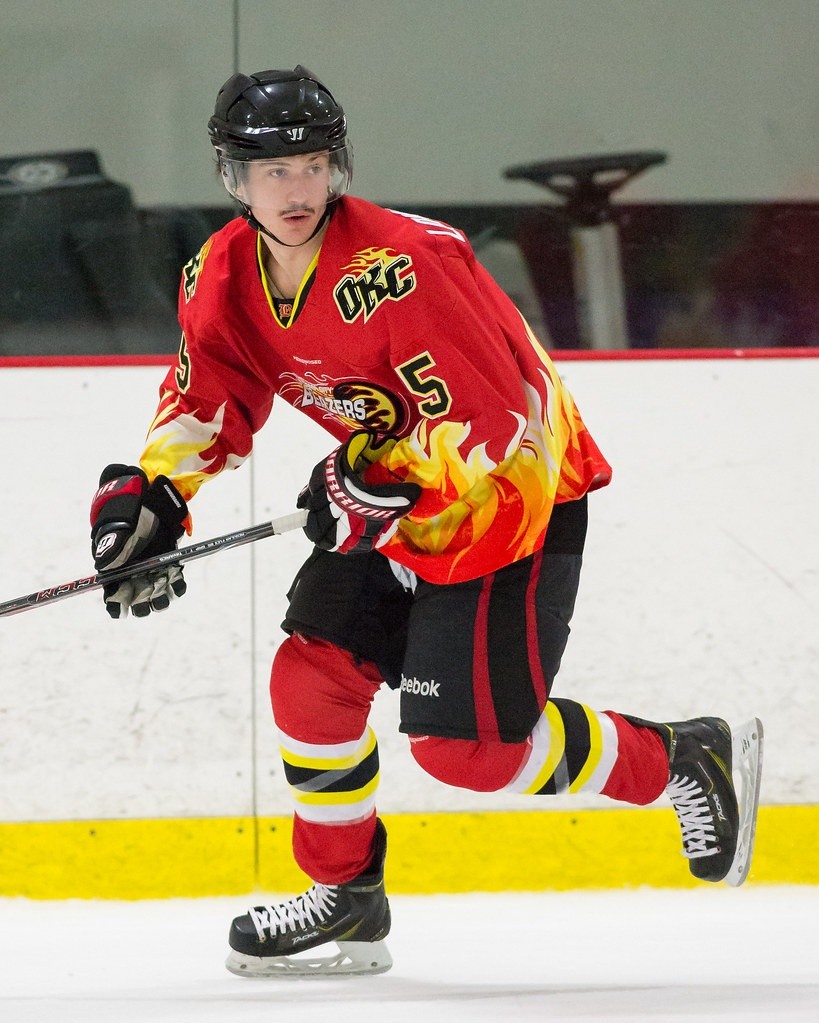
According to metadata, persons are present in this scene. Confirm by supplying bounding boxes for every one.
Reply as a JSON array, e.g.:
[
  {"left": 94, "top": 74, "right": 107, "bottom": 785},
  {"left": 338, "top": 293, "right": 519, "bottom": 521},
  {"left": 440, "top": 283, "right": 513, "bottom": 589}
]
[{"left": 89, "top": 65, "right": 766, "bottom": 977}]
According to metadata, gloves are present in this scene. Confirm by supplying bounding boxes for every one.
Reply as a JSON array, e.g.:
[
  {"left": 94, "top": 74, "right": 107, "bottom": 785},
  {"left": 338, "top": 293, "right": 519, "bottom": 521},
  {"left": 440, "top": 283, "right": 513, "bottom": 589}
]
[
  {"left": 295, "top": 428, "right": 423, "bottom": 555},
  {"left": 89, "top": 464, "right": 188, "bottom": 619}
]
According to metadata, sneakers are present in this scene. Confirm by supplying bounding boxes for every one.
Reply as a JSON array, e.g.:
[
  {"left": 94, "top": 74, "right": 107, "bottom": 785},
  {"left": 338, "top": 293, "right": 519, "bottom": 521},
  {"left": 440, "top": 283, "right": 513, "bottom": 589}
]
[
  {"left": 617, "top": 711, "right": 763, "bottom": 889},
  {"left": 224, "top": 816, "right": 392, "bottom": 978}
]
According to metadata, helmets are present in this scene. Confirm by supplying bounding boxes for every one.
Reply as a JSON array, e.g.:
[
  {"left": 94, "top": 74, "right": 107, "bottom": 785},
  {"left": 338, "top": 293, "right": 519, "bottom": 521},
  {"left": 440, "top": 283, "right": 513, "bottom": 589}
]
[{"left": 207, "top": 64, "right": 348, "bottom": 190}]
